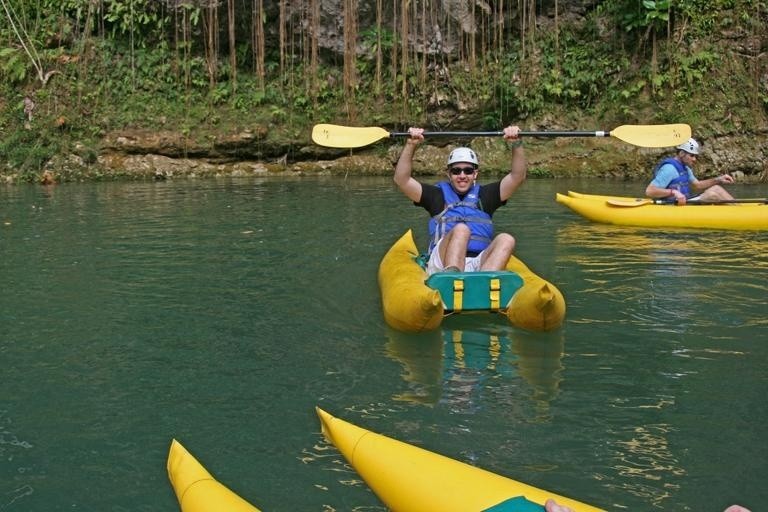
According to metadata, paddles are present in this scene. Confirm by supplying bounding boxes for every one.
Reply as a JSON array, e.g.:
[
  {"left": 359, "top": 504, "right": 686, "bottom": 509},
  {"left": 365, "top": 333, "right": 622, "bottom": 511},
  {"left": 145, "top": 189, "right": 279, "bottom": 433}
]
[
  {"left": 607, "top": 198, "right": 768, "bottom": 206},
  {"left": 312, "top": 123, "right": 692, "bottom": 148}
]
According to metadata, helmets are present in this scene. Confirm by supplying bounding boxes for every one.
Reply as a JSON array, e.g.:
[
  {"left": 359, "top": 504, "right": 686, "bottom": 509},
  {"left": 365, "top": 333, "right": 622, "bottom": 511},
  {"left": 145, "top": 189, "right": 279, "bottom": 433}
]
[
  {"left": 676, "top": 138, "right": 698, "bottom": 156},
  {"left": 448, "top": 147, "right": 478, "bottom": 166}
]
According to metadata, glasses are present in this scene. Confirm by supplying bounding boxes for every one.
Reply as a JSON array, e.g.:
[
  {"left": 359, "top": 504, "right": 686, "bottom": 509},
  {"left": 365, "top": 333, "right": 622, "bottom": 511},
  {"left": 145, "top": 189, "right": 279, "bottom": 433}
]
[{"left": 452, "top": 167, "right": 474, "bottom": 175}]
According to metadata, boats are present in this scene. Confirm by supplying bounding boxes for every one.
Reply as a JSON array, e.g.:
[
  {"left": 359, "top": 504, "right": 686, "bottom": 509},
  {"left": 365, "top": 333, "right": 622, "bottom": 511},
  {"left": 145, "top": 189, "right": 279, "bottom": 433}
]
[
  {"left": 377, "top": 227, "right": 569, "bottom": 335},
  {"left": 165, "top": 404, "right": 608, "bottom": 512},
  {"left": 554, "top": 190, "right": 768, "bottom": 235}
]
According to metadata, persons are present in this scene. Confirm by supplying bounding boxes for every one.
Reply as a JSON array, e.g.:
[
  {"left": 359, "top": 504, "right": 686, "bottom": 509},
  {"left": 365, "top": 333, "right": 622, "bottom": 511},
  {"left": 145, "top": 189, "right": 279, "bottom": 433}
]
[
  {"left": 393, "top": 125, "right": 528, "bottom": 276},
  {"left": 645, "top": 138, "right": 736, "bottom": 206}
]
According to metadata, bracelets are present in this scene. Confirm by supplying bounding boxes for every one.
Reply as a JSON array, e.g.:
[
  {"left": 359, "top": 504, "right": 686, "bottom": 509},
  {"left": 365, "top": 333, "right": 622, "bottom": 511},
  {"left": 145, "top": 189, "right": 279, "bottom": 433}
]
[
  {"left": 717, "top": 176, "right": 721, "bottom": 185},
  {"left": 671, "top": 188, "right": 673, "bottom": 196},
  {"left": 511, "top": 139, "right": 522, "bottom": 147}
]
